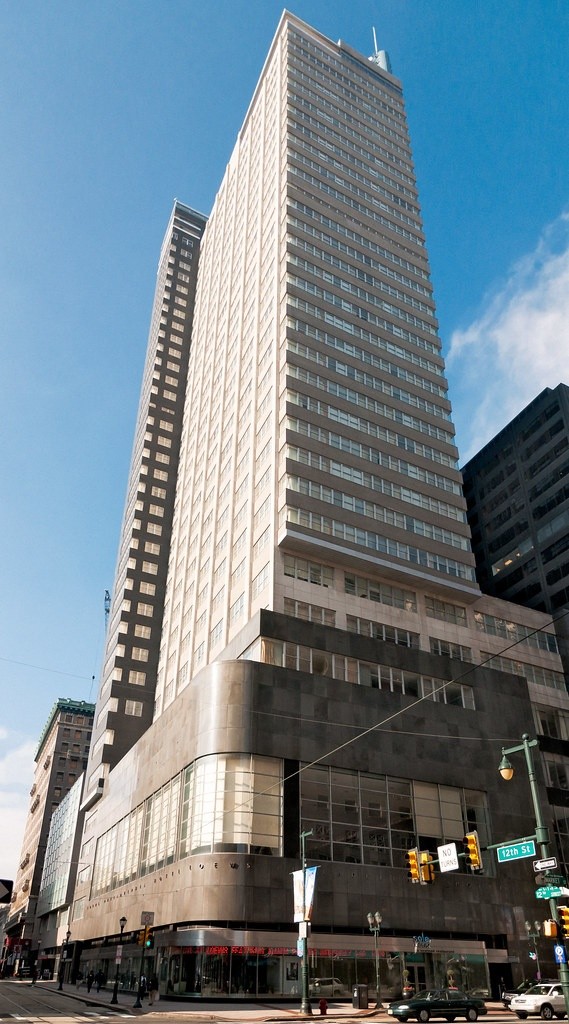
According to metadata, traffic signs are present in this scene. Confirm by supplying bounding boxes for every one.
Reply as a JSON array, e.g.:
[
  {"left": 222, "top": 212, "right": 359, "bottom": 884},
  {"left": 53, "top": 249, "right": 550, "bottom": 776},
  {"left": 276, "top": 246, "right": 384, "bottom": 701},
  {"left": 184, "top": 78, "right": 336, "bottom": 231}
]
[
  {"left": 532, "top": 857, "right": 557, "bottom": 872},
  {"left": 535, "top": 874, "right": 567, "bottom": 899}
]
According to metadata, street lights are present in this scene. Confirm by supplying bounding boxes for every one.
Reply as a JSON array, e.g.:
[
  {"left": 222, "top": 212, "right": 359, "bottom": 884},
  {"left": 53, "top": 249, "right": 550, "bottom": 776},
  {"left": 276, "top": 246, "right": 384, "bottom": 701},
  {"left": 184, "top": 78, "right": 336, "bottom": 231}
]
[
  {"left": 525, "top": 921, "right": 540, "bottom": 981},
  {"left": 57, "top": 931, "right": 72, "bottom": 991},
  {"left": 498, "top": 734, "right": 569, "bottom": 1019},
  {"left": 19, "top": 938, "right": 41, "bottom": 984},
  {"left": 110, "top": 917, "right": 127, "bottom": 1004},
  {"left": 367, "top": 912, "right": 383, "bottom": 1008}
]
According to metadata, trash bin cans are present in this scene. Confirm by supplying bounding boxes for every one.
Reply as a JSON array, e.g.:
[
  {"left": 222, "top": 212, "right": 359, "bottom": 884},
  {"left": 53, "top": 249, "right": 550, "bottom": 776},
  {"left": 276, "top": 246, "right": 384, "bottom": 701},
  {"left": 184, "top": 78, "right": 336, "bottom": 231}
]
[{"left": 352, "top": 983, "right": 369, "bottom": 1009}]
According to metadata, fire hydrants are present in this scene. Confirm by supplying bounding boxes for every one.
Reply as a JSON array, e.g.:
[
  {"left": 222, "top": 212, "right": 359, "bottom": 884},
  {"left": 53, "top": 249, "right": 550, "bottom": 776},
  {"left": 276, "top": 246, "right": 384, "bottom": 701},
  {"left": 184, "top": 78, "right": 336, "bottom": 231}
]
[{"left": 319, "top": 1000, "right": 328, "bottom": 1015}]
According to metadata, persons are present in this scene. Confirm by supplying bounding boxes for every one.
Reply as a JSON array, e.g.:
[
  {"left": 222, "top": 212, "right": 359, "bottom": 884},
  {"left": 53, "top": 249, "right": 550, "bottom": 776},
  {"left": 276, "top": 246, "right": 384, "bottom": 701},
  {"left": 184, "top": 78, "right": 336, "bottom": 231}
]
[
  {"left": 74, "top": 971, "right": 83, "bottom": 990},
  {"left": 86, "top": 970, "right": 95, "bottom": 993},
  {"left": 498, "top": 976, "right": 506, "bottom": 1002},
  {"left": 140, "top": 973, "right": 158, "bottom": 1006},
  {"left": 94, "top": 969, "right": 105, "bottom": 994},
  {"left": 114, "top": 970, "right": 136, "bottom": 991}
]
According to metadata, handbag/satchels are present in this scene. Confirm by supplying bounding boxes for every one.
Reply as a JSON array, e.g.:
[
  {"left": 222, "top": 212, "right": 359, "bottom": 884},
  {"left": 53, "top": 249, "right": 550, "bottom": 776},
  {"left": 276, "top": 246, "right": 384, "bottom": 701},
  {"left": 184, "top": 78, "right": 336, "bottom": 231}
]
[
  {"left": 155, "top": 991, "right": 160, "bottom": 1001},
  {"left": 147, "top": 985, "right": 152, "bottom": 991}
]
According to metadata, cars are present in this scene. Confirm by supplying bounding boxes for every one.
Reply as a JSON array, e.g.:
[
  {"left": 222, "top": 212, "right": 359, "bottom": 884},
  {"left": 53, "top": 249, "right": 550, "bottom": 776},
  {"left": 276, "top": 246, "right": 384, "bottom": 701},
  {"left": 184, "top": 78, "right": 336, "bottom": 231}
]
[
  {"left": 469, "top": 987, "right": 488, "bottom": 998},
  {"left": 502, "top": 979, "right": 549, "bottom": 1008},
  {"left": 388, "top": 989, "right": 488, "bottom": 1023},
  {"left": 511, "top": 984, "right": 567, "bottom": 1020}
]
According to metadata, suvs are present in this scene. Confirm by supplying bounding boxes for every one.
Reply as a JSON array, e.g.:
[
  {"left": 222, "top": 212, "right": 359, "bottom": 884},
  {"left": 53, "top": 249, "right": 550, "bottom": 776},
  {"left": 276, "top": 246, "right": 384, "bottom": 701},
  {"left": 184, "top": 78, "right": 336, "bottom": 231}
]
[{"left": 291, "top": 978, "right": 344, "bottom": 997}]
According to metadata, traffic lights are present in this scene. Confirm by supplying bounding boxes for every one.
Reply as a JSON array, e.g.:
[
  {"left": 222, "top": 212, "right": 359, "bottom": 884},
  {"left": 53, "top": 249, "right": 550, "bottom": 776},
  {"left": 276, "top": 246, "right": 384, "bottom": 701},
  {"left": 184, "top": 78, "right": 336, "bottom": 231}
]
[
  {"left": 404, "top": 847, "right": 419, "bottom": 880},
  {"left": 463, "top": 830, "right": 483, "bottom": 872},
  {"left": 145, "top": 928, "right": 153, "bottom": 950},
  {"left": 556, "top": 908, "right": 567, "bottom": 936},
  {"left": 138, "top": 931, "right": 144, "bottom": 947},
  {"left": 418, "top": 851, "right": 434, "bottom": 880},
  {"left": 560, "top": 908, "right": 569, "bottom": 937}
]
